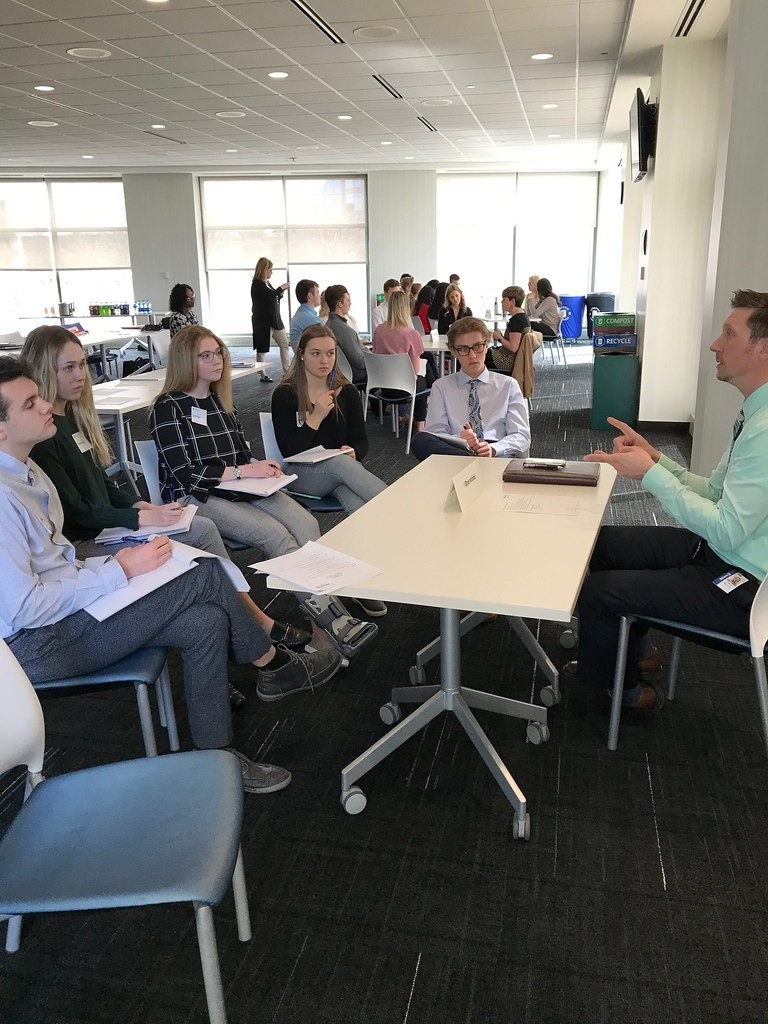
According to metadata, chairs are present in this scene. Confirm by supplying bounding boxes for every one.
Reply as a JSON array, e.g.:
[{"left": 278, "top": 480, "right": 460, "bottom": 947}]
[
  {"left": 149, "top": 331, "right": 172, "bottom": 368},
  {"left": 607, "top": 572, "right": 768, "bottom": 751},
  {"left": 336, "top": 310, "right": 568, "bottom": 456},
  {"left": 1, "top": 411, "right": 344, "bottom": 1024}
]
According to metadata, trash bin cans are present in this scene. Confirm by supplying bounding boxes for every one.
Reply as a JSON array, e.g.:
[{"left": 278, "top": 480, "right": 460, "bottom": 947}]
[
  {"left": 587, "top": 292, "right": 616, "bottom": 339},
  {"left": 558, "top": 294, "right": 585, "bottom": 338}
]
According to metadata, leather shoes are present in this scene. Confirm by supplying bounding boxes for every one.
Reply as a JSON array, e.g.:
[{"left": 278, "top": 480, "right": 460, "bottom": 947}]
[
  {"left": 604, "top": 683, "right": 664, "bottom": 714},
  {"left": 269, "top": 619, "right": 311, "bottom": 647},
  {"left": 227, "top": 683, "right": 247, "bottom": 706},
  {"left": 563, "top": 646, "right": 664, "bottom": 680}
]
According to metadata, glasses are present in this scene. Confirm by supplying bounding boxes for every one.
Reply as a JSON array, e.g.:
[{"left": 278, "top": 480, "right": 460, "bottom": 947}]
[
  {"left": 198, "top": 346, "right": 226, "bottom": 363},
  {"left": 453, "top": 339, "right": 487, "bottom": 356}
]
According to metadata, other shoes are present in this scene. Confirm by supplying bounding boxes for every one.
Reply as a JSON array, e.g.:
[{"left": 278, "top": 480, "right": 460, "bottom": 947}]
[
  {"left": 260, "top": 375, "right": 272, "bottom": 383},
  {"left": 353, "top": 597, "right": 388, "bottom": 615},
  {"left": 400, "top": 414, "right": 414, "bottom": 423}
]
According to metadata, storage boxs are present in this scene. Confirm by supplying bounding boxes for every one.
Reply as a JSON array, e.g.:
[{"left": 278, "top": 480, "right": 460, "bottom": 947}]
[{"left": 593, "top": 312, "right": 638, "bottom": 356}]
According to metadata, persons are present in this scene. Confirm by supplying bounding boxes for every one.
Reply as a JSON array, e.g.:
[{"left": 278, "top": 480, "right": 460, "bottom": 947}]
[
  {"left": 319, "top": 285, "right": 392, "bottom": 417},
  {"left": 524, "top": 276, "right": 539, "bottom": 317},
  {"left": 372, "top": 273, "right": 472, "bottom": 436},
  {"left": 271, "top": 324, "right": 390, "bottom": 616},
  {"left": 526, "top": 278, "right": 562, "bottom": 341},
  {"left": 251, "top": 257, "right": 291, "bottom": 382},
  {"left": 0, "top": 354, "right": 344, "bottom": 794},
  {"left": 169, "top": 283, "right": 198, "bottom": 340},
  {"left": 147, "top": 327, "right": 379, "bottom": 668},
  {"left": 411, "top": 317, "right": 531, "bottom": 623},
  {"left": 728, "top": 577, "right": 741, "bottom": 586},
  {"left": 289, "top": 280, "right": 325, "bottom": 355},
  {"left": 17, "top": 326, "right": 314, "bottom": 715},
  {"left": 562, "top": 290, "right": 768, "bottom": 711},
  {"left": 483, "top": 286, "right": 531, "bottom": 378}
]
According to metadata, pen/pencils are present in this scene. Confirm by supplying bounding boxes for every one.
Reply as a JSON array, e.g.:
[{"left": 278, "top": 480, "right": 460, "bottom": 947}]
[
  {"left": 523, "top": 463, "right": 563, "bottom": 469},
  {"left": 170, "top": 482, "right": 175, "bottom": 511},
  {"left": 329, "top": 370, "right": 336, "bottom": 390},
  {"left": 121, "top": 536, "right": 149, "bottom": 544}
]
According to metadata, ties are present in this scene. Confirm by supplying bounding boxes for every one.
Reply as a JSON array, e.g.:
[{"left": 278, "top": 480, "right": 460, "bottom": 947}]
[
  {"left": 467, "top": 379, "right": 484, "bottom": 443},
  {"left": 720, "top": 409, "right": 745, "bottom": 499}
]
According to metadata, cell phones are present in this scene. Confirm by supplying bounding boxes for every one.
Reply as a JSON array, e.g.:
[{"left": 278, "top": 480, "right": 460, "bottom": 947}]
[{"left": 523, "top": 458, "right": 566, "bottom": 469}]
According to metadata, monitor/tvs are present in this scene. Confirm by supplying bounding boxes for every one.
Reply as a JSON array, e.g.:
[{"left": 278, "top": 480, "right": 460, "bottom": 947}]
[{"left": 628, "top": 88, "right": 657, "bottom": 182}]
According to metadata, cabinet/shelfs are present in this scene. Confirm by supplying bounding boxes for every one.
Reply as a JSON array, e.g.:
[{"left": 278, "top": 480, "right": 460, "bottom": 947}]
[{"left": 590, "top": 352, "right": 640, "bottom": 431}]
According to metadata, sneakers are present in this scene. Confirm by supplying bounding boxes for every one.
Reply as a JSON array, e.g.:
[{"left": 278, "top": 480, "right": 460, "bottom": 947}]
[
  {"left": 192, "top": 747, "right": 292, "bottom": 792},
  {"left": 256, "top": 644, "right": 342, "bottom": 701}
]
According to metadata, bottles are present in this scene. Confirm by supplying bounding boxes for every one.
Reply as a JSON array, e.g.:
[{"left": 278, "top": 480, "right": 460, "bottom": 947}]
[
  {"left": 485, "top": 309, "right": 491, "bottom": 319},
  {"left": 89, "top": 300, "right": 153, "bottom": 316},
  {"left": 493, "top": 296, "right": 498, "bottom": 315}
]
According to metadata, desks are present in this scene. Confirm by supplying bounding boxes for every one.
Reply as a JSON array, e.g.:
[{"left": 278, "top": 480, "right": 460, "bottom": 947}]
[
  {"left": 421, "top": 334, "right": 457, "bottom": 379},
  {"left": 473, "top": 311, "right": 543, "bottom": 348},
  {"left": 0, "top": 308, "right": 274, "bottom": 499},
  {"left": 265, "top": 454, "right": 618, "bottom": 843}
]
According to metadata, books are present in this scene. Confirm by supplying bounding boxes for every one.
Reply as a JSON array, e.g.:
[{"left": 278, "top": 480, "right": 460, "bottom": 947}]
[
  {"left": 231, "top": 362, "right": 255, "bottom": 368},
  {"left": 503, "top": 460, "right": 601, "bottom": 487}
]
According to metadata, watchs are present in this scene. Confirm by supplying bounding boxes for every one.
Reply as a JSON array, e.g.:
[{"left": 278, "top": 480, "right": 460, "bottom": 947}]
[{"left": 234, "top": 465, "right": 242, "bottom": 479}]
